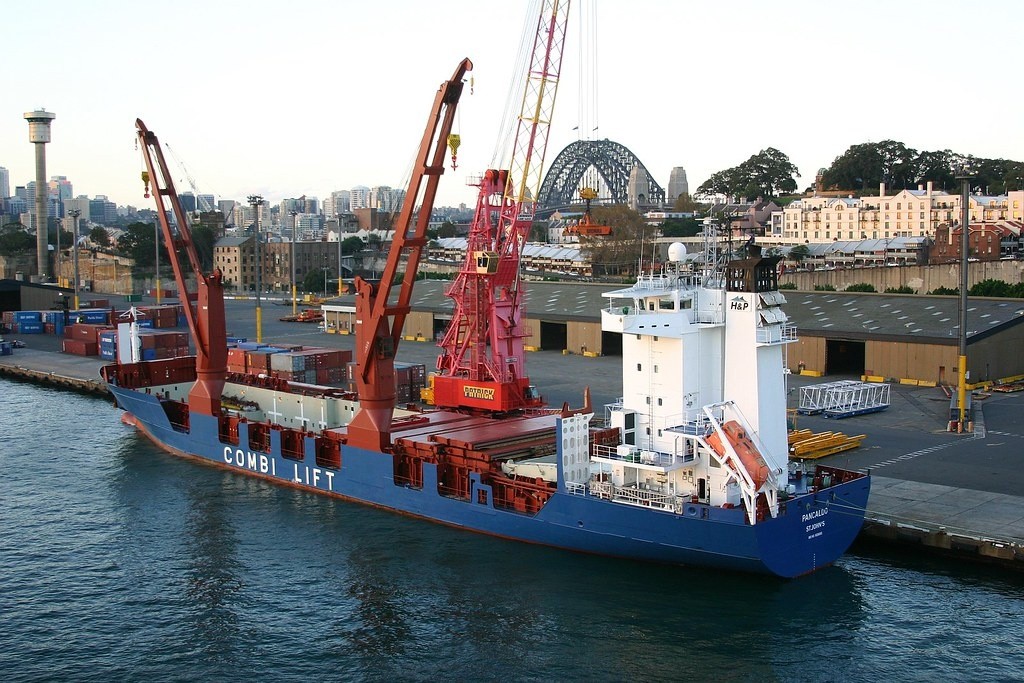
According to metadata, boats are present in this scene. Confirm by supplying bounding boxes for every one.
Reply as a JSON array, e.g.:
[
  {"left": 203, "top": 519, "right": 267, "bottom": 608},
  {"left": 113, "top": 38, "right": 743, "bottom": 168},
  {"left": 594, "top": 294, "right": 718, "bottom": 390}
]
[{"left": 98, "top": 0, "right": 872, "bottom": 578}]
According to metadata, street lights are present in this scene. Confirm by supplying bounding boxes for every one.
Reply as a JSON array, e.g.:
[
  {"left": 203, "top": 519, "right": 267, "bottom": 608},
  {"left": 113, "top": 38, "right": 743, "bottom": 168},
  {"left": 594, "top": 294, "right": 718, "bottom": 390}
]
[
  {"left": 150, "top": 210, "right": 162, "bottom": 304},
  {"left": 288, "top": 205, "right": 299, "bottom": 315},
  {"left": 954, "top": 168, "right": 978, "bottom": 423},
  {"left": 66, "top": 207, "right": 81, "bottom": 312},
  {"left": 333, "top": 210, "right": 347, "bottom": 297},
  {"left": 245, "top": 193, "right": 265, "bottom": 344}
]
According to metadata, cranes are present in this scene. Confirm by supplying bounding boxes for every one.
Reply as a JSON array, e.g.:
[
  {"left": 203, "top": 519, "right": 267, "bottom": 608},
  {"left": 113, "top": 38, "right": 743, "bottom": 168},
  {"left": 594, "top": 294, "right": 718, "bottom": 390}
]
[{"left": 419, "top": 0, "right": 616, "bottom": 419}]
